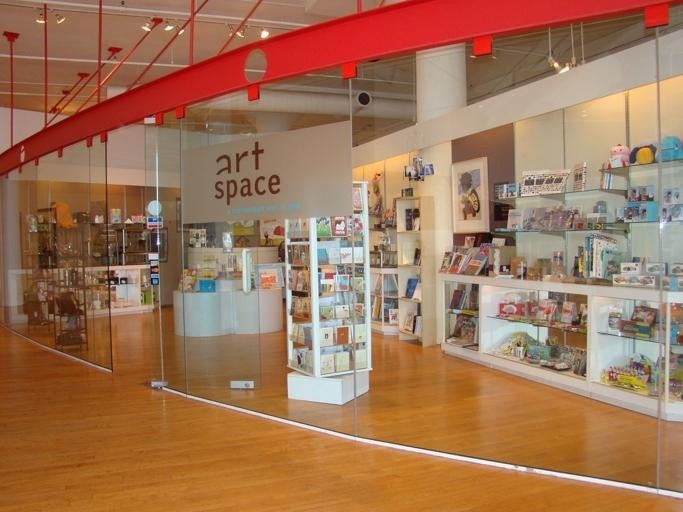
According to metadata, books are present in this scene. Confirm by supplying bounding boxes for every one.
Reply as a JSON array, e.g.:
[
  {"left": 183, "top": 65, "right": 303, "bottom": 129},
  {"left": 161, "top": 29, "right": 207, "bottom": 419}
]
[
  {"left": 572, "top": 232, "right": 624, "bottom": 281},
  {"left": 505, "top": 205, "right": 582, "bottom": 230},
  {"left": 370, "top": 273, "right": 422, "bottom": 336},
  {"left": 177, "top": 269, "right": 196, "bottom": 292},
  {"left": 438, "top": 235, "right": 506, "bottom": 275},
  {"left": 498, "top": 290, "right": 587, "bottom": 329},
  {"left": 286, "top": 183, "right": 369, "bottom": 376},
  {"left": 448, "top": 288, "right": 478, "bottom": 345},
  {"left": 518, "top": 161, "right": 590, "bottom": 194},
  {"left": 405, "top": 208, "right": 421, "bottom": 231}
]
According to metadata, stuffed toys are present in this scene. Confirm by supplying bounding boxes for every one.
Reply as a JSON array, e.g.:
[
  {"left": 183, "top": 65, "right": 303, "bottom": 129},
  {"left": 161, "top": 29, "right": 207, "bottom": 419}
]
[{"left": 607, "top": 134, "right": 683, "bottom": 169}]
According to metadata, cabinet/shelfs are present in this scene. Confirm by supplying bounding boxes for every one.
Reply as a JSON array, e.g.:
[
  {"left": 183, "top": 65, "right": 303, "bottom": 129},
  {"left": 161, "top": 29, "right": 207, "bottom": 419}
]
[
  {"left": 172, "top": 221, "right": 286, "bottom": 339},
  {"left": 368, "top": 158, "right": 682, "bottom": 424},
  {"left": 22, "top": 220, "right": 169, "bottom": 319},
  {"left": 284, "top": 181, "right": 373, "bottom": 406}
]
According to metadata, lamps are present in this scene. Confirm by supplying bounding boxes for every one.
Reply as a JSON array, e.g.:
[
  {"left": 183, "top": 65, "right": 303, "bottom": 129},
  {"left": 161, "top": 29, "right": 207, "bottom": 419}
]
[
  {"left": 37, "top": 8, "right": 269, "bottom": 40},
  {"left": 545, "top": 28, "right": 570, "bottom": 74}
]
